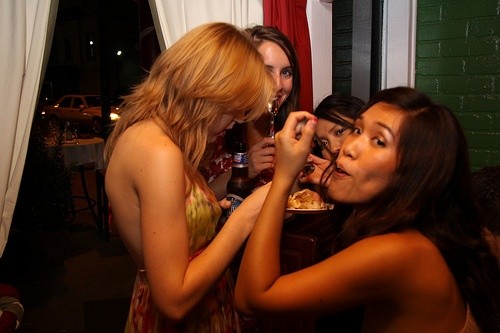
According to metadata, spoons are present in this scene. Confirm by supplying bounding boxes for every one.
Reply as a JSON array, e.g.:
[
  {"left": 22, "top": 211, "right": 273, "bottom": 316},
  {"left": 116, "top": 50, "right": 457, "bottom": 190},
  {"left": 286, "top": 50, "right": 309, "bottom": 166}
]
[{"left": 266, "top": 100, "right": 279, "bottom": 137}]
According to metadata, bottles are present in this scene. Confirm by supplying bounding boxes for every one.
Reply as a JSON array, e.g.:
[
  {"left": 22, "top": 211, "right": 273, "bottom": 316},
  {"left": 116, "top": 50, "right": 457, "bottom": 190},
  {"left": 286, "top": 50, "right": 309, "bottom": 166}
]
[{"left": 226, "top": 140, "right": 250, "bottom": 220}]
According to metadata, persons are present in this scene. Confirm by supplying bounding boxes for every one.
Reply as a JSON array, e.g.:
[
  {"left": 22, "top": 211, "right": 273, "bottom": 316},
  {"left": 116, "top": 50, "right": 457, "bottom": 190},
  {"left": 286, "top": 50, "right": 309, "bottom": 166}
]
[
  {"left": 198, "top": 26, "right": 302, "bottom": 213},
  {"left": 231, "top": 87, "right": 500, "bottom": 333},
  {"left": 307, "top": 93, "right": 370, "bottom": 161},
  {"left": 464, "top": 163, "right": 500, "bottom": 289},
  {"left": 102, "top": 21, "right": 300, "bottom": 333}
]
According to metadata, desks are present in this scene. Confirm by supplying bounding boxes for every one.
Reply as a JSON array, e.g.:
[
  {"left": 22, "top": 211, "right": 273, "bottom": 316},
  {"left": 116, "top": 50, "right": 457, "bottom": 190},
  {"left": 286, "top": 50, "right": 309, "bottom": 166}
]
[{"left": 45, "top": 137, "right": 104, "bottom": 229}]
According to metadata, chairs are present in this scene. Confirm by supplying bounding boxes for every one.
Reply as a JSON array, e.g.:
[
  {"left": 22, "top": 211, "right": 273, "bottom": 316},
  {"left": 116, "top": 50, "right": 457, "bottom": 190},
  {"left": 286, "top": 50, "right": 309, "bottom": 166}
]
[{"left": 96, "top": 169, "right": 111, "bottom": 242}]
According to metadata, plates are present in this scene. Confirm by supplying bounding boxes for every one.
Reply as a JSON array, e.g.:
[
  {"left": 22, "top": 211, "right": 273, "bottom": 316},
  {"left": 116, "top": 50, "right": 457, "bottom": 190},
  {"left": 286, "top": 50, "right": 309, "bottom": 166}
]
[{"left": 285, "top": 203, "right": 334, "bottom": 213}]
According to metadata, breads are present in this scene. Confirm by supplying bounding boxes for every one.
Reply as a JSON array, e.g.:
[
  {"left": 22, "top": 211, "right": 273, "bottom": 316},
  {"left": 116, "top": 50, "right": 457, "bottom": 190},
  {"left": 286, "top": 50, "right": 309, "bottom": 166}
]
[{"left": 287, "top": 189, "right": 325, "bottom": 210}]
